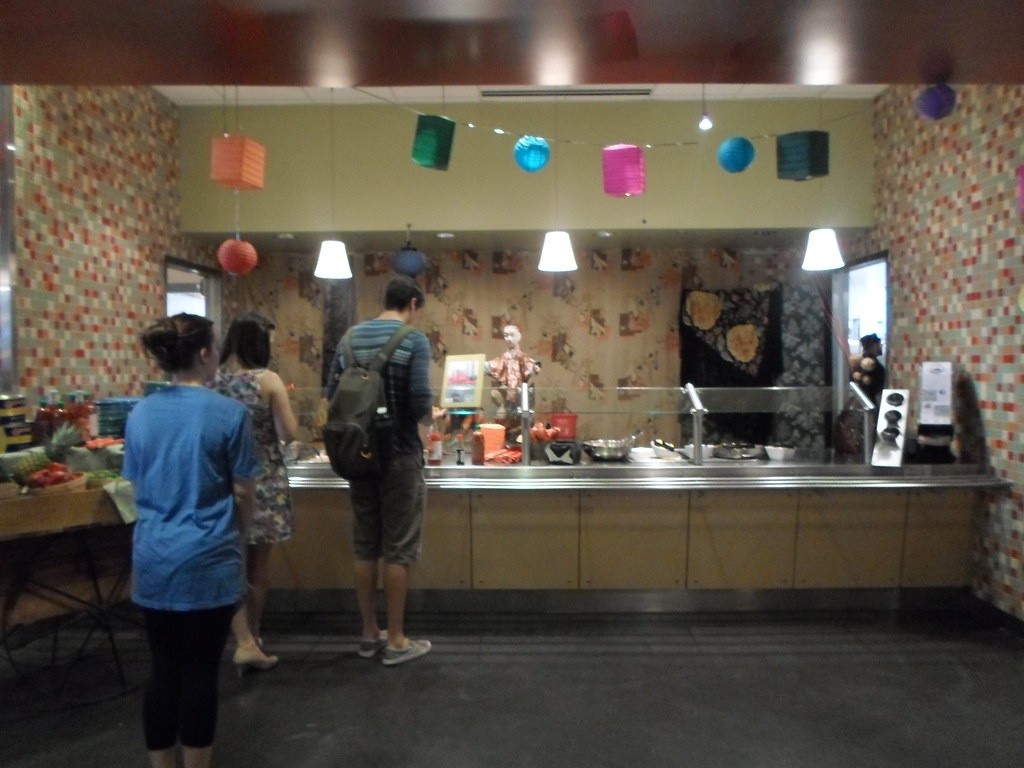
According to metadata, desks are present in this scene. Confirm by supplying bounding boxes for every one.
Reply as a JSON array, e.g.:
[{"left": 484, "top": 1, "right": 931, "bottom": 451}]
[{"left": 0, "top": 481, "right": 137, "bottom": 709}]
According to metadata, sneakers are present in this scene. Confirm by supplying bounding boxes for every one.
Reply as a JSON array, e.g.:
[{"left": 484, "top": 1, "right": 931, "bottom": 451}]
[
  {"left": 358, "top": 629, "right": 388, "bottom": 657},
  {"left": 382, "top": 639, "right": 433, "bottom": 664}
]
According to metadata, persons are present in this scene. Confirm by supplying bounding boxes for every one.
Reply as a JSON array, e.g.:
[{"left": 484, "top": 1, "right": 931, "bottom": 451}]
[
  {"left": 122, "top": 311, "right": 265, "bottom": 768},
  {"left": 858, "top": 334, "right": 886, "bottom": 401},
  {"left": 483, "top": 324, "right": 540, "bottom": 437},
  {"left": 321, "top": 279, "right": 448, "bottom": 663},
  {"left": 203, "top": 311, "right": 298, "bottom": 675}
]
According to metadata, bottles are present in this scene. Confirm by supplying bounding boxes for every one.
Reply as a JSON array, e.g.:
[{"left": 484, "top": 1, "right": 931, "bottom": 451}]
[
  {"left": 456, "top": 434, "right": 464, "bottom": 465},
  {"left": 33, "top": 390, "right": 98, "bottom": 447},
  {"left": 427, "top": 431, "right": 442, "bottom": 466}
]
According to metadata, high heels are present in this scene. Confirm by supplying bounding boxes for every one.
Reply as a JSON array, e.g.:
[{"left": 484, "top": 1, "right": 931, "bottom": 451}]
[{"left": 234, "top": 638, "right": 279, "bottom": 680}]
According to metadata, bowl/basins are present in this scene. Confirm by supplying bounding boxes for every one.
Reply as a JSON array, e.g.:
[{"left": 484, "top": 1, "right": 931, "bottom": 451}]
[
  {"left": 684, "top": 443, "right": 715, "bottom": 458},
  {"left": 764, "top": 445, "right": 797, "bottom": 461},
  {"left": 582, "top": 439, "right": 634, "bottom": 461}
]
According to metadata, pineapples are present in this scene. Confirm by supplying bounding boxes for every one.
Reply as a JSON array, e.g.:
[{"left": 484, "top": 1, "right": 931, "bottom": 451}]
[{"left": 10, "top": 421, "right": 81, "bottom": 486}]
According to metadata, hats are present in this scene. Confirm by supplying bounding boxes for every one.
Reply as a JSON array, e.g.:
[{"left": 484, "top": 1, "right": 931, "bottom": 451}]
[{"left": 860, "top": 334, "right": 881, "bottom": 348}]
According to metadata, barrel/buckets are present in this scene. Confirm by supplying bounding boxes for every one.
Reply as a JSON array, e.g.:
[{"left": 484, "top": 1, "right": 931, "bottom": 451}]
[{"left": 552, "top": 414, "right": 577, "bottom": 440}]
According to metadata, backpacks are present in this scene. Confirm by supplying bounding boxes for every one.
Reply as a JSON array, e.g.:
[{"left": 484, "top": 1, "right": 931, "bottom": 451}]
[{"left": 321, "top": 325, "right": 416, "bottom": 479}]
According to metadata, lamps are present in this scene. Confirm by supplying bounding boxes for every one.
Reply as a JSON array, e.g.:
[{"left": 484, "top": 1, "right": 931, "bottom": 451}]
[
  {"left": 801, "top": 229, "right": 845, "bottom": 271},
  {"left": 313, "top": 240, "right": 352, "bottom": 279},
  {"left": 537, "top": 231, "right": 577, "bottom": 271}
]
